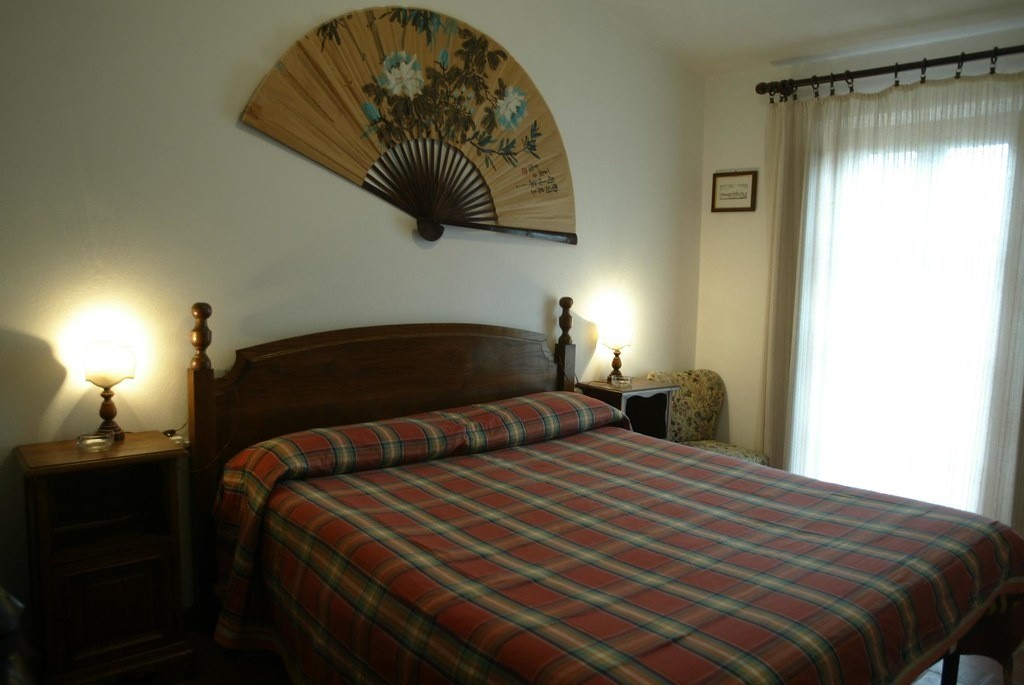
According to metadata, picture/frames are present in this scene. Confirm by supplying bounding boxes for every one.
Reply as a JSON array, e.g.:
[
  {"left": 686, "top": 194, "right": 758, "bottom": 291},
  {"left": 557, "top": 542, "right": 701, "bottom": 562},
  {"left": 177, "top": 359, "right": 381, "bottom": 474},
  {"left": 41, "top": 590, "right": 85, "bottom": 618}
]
[{"left": 711, "top": 170, "right": 758, "bottom": 212}]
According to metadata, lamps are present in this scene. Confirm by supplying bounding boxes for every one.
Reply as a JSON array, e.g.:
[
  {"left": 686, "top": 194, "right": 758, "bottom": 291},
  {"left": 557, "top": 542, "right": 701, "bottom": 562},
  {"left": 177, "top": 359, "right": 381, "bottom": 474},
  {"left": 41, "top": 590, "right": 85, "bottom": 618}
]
[
  {"left": 585, "top": 307, "right": 636, "bottom": 384},
  {"left": 59, "top": 305, "right": 153, "bottom": 443}
]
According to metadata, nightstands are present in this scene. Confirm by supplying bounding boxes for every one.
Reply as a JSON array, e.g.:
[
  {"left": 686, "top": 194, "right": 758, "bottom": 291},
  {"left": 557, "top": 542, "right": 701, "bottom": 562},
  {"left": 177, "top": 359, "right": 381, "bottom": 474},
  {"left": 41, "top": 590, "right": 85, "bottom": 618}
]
[
  {"left": 13, "top": 427, "right": 194, "bottom": 685},
  {"left": 575, "top": 377, "right": 681, "bottom": 442}
]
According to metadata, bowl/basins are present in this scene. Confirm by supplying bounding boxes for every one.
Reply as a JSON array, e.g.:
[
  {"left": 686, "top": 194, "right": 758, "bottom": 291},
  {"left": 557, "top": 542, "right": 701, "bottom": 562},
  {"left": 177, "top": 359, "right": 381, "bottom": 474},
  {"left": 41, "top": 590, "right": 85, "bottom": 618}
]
[
  {"left": 611, "top": 375, "right": 631, "bottom": 388},
  {"left": 77, "top": 430, "right": 113, "bottom": 453}
]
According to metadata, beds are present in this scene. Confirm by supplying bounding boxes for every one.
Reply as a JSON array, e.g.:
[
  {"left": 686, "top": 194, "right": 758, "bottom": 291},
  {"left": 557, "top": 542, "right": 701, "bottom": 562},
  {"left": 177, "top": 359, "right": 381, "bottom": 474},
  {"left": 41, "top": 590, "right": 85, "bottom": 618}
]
[{"left": 187, "top": 296, "right": 1024, "bottom": 685}]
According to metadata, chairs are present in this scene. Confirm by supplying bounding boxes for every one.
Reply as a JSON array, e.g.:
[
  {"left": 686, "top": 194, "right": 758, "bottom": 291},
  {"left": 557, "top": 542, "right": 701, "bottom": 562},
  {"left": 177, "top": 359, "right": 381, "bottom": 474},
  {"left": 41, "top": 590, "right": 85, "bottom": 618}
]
[{"left": 640, "top": 369, "right": 771, "bottom": 467}]
